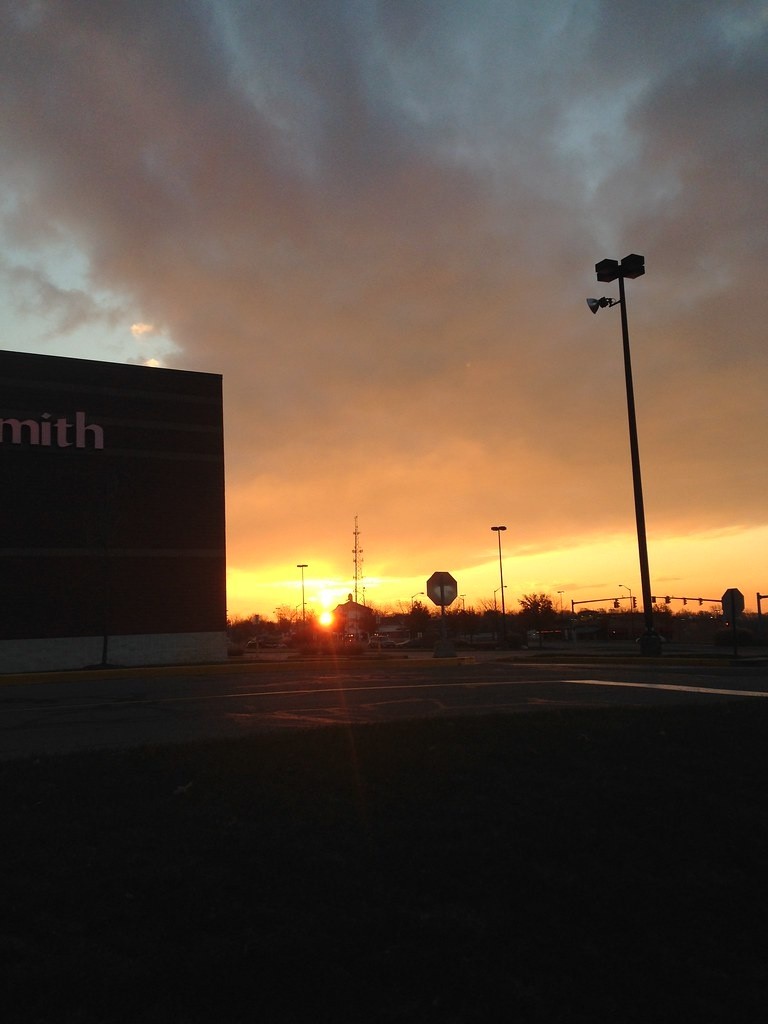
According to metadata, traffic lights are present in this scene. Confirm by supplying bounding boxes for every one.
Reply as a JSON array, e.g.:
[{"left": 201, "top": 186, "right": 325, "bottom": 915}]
[
  {"left": 666, "top": 596, "right": 671, "bottom": 603},
  {"left": 633, "top": 597, "right": 637, "bottom": 608},
  {"left": 614, "top": 602, "right": 620, "bottom": 608},
  {"left": 684, "top": 599, "right": 687, "bottom": 605},
  {"left": 699, "top": 598, "right": 703, "bottom": 605}
]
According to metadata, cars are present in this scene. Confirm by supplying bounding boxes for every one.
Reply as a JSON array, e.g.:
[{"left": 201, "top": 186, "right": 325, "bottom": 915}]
[
  {"left": 369, "top": 636, "right": 395, "bottom": 648},
  {"left": 636, "top": 635, "right": 665, "bottom": 644}
]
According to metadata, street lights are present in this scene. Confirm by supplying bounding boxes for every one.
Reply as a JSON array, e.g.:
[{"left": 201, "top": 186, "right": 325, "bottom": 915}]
[
  {"left": 618, "top": 584, "right": 632, "bottom": 613},
  {"left": 296, "top": 603, "right": 307, "bottom": 624},
  {"left": 587, "top": 253, "right": 662, "bottom": 654},
  {"left": 411, "top": 592, "right": 425, "bottom": 612},
  {"left": 494, "top": 586, "right": 507, "bottom": 613},
  {"left": 298, "top": 565, "right": 308, "bottom": 636},
  {"left": 558, "top": 590, "right": 565, "bottom": 627},
  {"left": 492, "top": 525, "right": 512, "bottom": 638}
]
